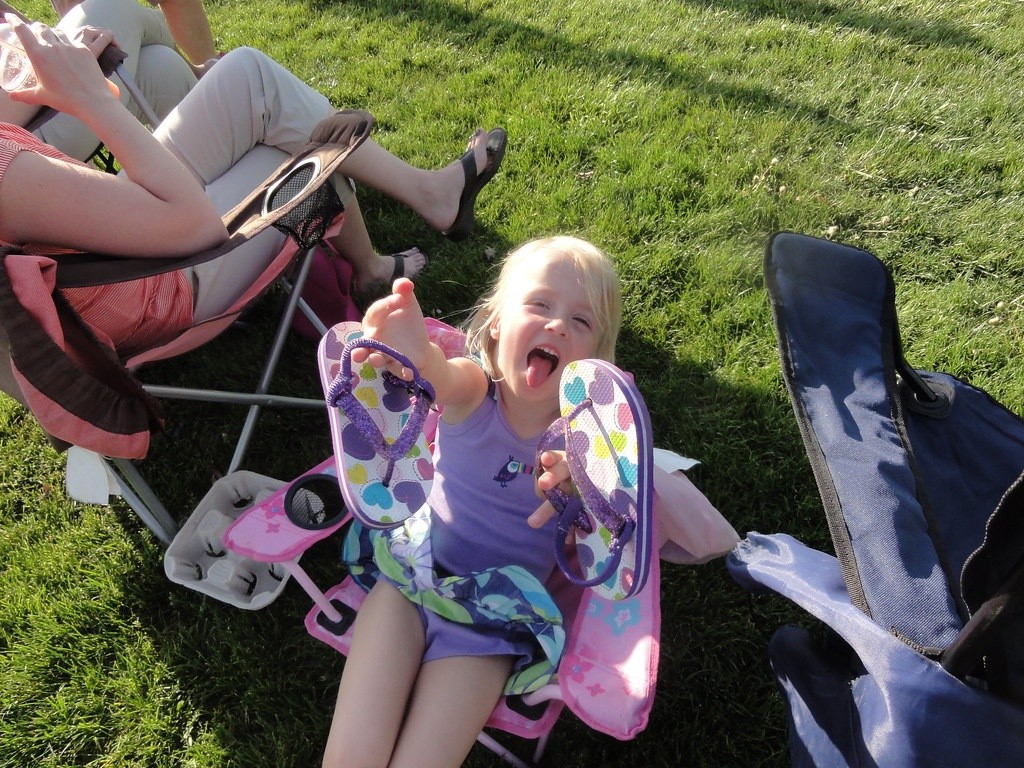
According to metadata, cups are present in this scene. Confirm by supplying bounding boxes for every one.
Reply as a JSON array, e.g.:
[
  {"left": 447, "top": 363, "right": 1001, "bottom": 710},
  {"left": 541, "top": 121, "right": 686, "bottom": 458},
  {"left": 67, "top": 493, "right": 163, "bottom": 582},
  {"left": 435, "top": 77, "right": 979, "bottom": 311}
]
[{"left": 0, "top": 21, "right": 122, "bottom": 102}]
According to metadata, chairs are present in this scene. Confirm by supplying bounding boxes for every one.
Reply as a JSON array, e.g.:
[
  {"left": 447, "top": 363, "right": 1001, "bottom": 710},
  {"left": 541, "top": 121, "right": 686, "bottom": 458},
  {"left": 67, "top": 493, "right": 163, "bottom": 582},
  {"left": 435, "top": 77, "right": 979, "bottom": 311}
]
[
  {"left": 0, "top": 109, "right": 379, "bottom": 554},
  {"left": 220, "top": 449, "right": 588, "bottom": 766}
]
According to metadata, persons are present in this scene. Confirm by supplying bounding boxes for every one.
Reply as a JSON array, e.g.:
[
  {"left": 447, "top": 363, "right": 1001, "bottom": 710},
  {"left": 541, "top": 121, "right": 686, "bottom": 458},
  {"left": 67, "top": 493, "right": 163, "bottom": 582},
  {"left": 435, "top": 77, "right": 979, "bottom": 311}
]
[
  {"left": 0, "top": 0, "right": 220, "bottom": 168},
  {"left": 157, "top": 0, "right": 222, "bottom": 66},
  {"left": 319, "top": 235, "right": 622, "bottom": 768},
  {"left": 0, "top": 12, "right": 491, "bottom": 358}
]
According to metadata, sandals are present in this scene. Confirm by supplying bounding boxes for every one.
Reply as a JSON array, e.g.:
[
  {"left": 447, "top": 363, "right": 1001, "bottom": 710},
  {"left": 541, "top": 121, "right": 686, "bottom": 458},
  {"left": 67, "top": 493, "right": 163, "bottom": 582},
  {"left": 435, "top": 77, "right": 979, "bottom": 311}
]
[
  {"left": 439, "top": 127, "right": 508, "bottom": 245},
  {"left": 315, "top": 320, "right": 435, "bottom": 530},
  {"left": 534, "top": 357, "right": 651, "bottom": 599},
  {"left": 356, "top": 246, "right": 432, "bottom": 307}
]
